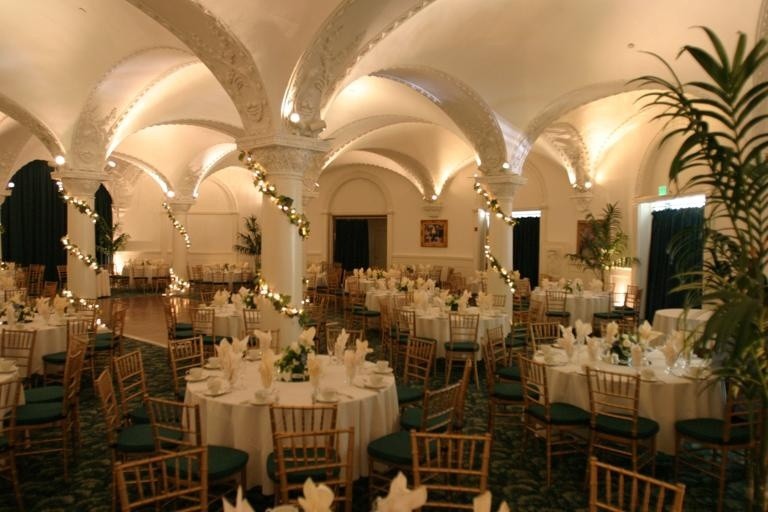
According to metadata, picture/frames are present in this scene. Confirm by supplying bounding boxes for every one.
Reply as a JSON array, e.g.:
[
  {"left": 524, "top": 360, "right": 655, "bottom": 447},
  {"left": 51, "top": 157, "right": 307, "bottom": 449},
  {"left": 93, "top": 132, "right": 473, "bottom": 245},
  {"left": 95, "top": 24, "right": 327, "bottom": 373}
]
[{"left": 420, "top": 219, "right": 448, "bottom": 248}]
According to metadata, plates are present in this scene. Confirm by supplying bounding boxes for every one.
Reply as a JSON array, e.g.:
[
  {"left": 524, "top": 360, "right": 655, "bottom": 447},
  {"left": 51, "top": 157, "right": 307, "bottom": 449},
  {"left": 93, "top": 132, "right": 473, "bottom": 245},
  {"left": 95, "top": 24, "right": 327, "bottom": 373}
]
[
  {"left": 537, "top": 338, "right": 715, "bottom": 381},
  {"left": 185, "top": 347, "right": 393, "bottom": 403}
]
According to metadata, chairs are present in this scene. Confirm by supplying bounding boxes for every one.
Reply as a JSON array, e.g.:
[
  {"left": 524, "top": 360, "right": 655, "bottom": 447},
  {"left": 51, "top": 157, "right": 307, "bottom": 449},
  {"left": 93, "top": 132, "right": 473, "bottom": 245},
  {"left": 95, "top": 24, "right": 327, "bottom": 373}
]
[
  {"left": 111, "top": 443, "right": 211, "bottom": 512},
  {"left": 160, "top": 254, "right": 735, "bottom": 435},
  {"left": 408, "top": 429, "right": 493, "bottom": 512},
  {"left": 0, "top": 250, "right": 180, "bottom": 510},
  {"left": 266, "top": 401, "right": 344, "bottom": 503},
  {"left": 581, "top": 362, "right": 661, "bottom": 487},
  {"left": 364, "top": 378, "right": 466, "bottom": 504},
  {"left": 274, "top": 425, "right": 357, "bottom": 512},
  {"left": 143, "top": 392, "right": 252, "bottom": 504},
  {"left": 515, "top": 351, "right": 591, "bottom": 488},
  {"left": 584, "top": 455, "right": 689, "bottom": 510},
  {"left": 671, "top": 379, "right": 768, "bottom": 510}
]
